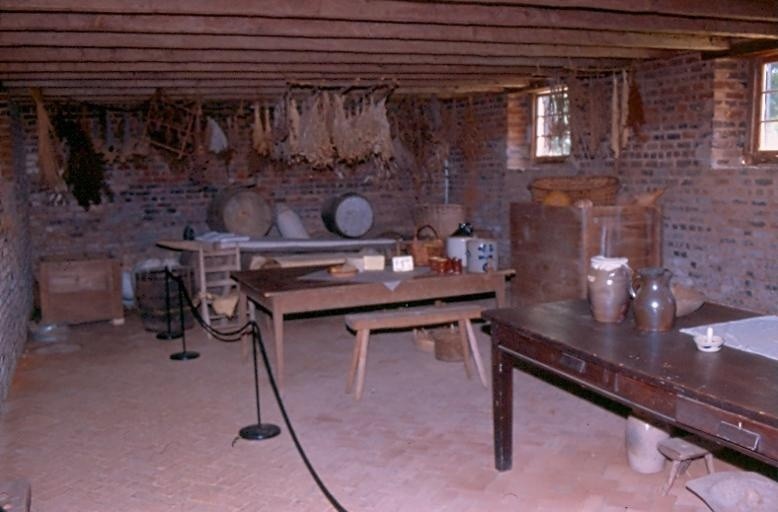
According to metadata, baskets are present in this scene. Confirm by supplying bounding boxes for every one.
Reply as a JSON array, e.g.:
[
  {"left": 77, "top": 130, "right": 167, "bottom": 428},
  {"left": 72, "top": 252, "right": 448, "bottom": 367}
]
[
  {"left": 411, "top": 225, "right": 444, "bottom": 266},
  {"left": 410, "top": 204, "right": 466, "bottom": 237},
  {"left": 526, "top": 175, "right": 620, "bottom": 208}
]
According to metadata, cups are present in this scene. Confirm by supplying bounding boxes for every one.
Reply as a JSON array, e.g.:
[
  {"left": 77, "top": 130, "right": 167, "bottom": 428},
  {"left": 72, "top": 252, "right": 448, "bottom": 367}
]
[{"left": 429, "top": 255, "right": 463, "bottom": 275}]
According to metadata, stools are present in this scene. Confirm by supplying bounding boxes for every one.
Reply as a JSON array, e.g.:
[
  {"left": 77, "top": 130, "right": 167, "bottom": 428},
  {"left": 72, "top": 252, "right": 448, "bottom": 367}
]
[{"left": 346, "top": 297, "right": 488, "bottom": 400}]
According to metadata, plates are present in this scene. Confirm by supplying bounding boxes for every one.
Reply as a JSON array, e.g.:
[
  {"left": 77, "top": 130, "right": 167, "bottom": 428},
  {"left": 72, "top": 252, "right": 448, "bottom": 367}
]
[{"left": 327, "top": 265, "right": 359, "bottom": 279}]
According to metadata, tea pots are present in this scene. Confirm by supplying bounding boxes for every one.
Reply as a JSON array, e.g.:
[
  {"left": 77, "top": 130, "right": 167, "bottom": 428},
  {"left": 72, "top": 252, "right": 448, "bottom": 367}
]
[{"left": 581, "top": 257, "right": 677, "bottom": 331}]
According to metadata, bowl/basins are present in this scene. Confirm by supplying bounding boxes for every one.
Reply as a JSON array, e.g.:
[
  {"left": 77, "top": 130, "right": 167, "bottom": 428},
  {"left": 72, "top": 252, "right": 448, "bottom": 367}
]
[{"left": 694, "top": 335, "right": 724, "bottom": 352}]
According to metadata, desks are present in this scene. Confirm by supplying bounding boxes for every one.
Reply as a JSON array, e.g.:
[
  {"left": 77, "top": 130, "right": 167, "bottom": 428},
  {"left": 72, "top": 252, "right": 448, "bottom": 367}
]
[
  {"left": 480, "top": 300, "right": 777, "bottom": 472},
  {"left": 154, "top": 234, "right": 413, "bottom": 319},
  {"left": 229, "top": 264, "right": 516, "bottom": 380}
]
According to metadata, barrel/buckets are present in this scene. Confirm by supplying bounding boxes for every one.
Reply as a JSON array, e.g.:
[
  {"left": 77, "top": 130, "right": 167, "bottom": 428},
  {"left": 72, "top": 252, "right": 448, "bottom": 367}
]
[
  {"left": 208, "top": 183, "right": 273, "bottom": 239},
  {"left": 136, "top": 266, "right": 193, "bottom": 334},
  {"left": 448, "top": 236, "right": 472, "bottom": 265},
  {"left": 322, "top": 191, "right": 375, "bottom": 239}
]
